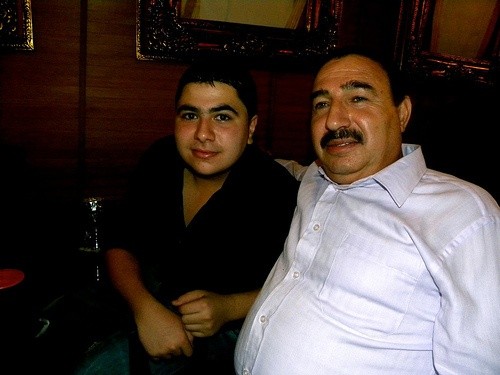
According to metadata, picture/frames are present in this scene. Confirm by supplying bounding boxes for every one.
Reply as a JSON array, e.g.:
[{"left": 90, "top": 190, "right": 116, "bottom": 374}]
[
  {"left": 135, "top": 0, "right": 344, "bottom": 78},
  {"left": 0, "top": 0, "right": 37, "bottom": 51},
  {"left": 392, "top": 0, "right": 499, "bottom": 93}
]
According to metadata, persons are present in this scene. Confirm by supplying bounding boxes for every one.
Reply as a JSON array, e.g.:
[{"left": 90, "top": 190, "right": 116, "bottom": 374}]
[
  {"left": 104, "top": 58, "right": 303, "bottom": 375},
  {"left": 231, "top": 41, "right": 500, "bottom": 375}
]
